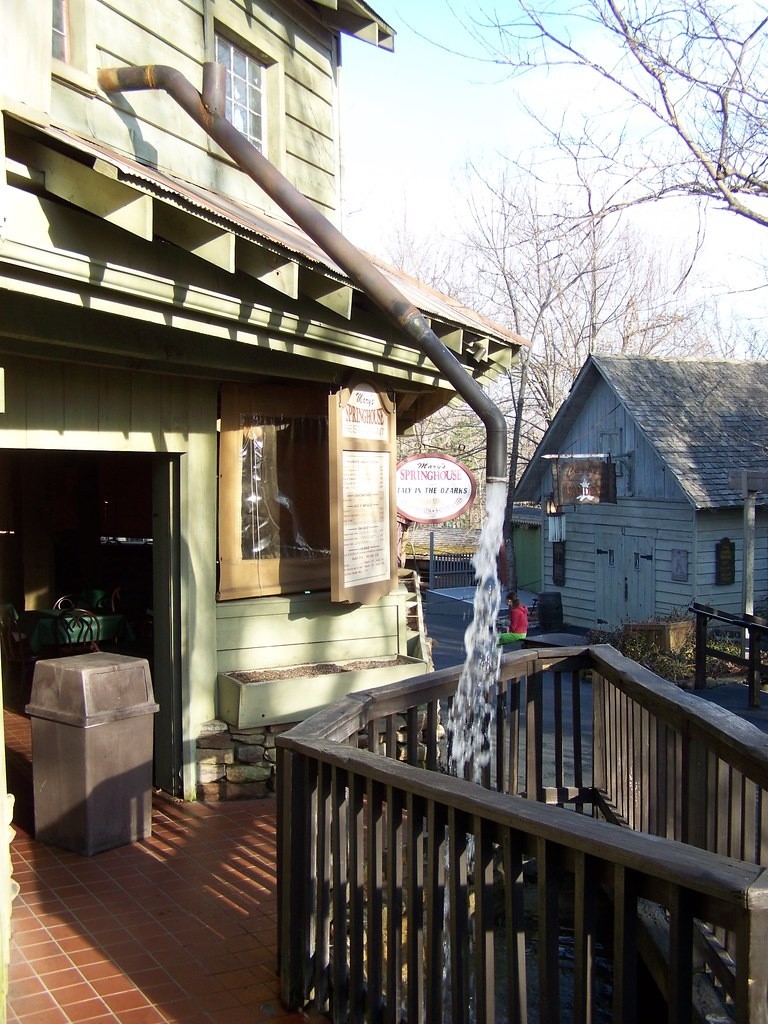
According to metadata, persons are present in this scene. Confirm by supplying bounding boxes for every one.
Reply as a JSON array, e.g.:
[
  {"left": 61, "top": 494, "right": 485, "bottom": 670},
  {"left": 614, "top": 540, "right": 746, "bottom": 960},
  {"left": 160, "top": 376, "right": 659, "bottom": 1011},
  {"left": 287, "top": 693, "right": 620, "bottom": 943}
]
[{"left": 496, "top": 592, "right": 528, "bottom": 647}]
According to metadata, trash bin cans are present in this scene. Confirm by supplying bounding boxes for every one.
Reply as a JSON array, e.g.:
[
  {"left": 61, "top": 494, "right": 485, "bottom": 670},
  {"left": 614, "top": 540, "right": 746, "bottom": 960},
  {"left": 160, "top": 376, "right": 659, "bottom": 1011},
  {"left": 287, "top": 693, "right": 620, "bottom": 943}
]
[
  {"left": 25, "top": 651, "right": 160, "bottom": 865},
  {"left": 537, "top": 592, "right": 564, "bottom": 630}
]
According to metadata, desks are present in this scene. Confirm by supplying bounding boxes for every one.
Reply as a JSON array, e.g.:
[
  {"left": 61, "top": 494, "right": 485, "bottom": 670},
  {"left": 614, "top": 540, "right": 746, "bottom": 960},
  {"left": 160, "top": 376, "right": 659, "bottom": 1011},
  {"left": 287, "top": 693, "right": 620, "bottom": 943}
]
[{"left": 520, "top": 634, "right": 591, "bottom": 649}]
